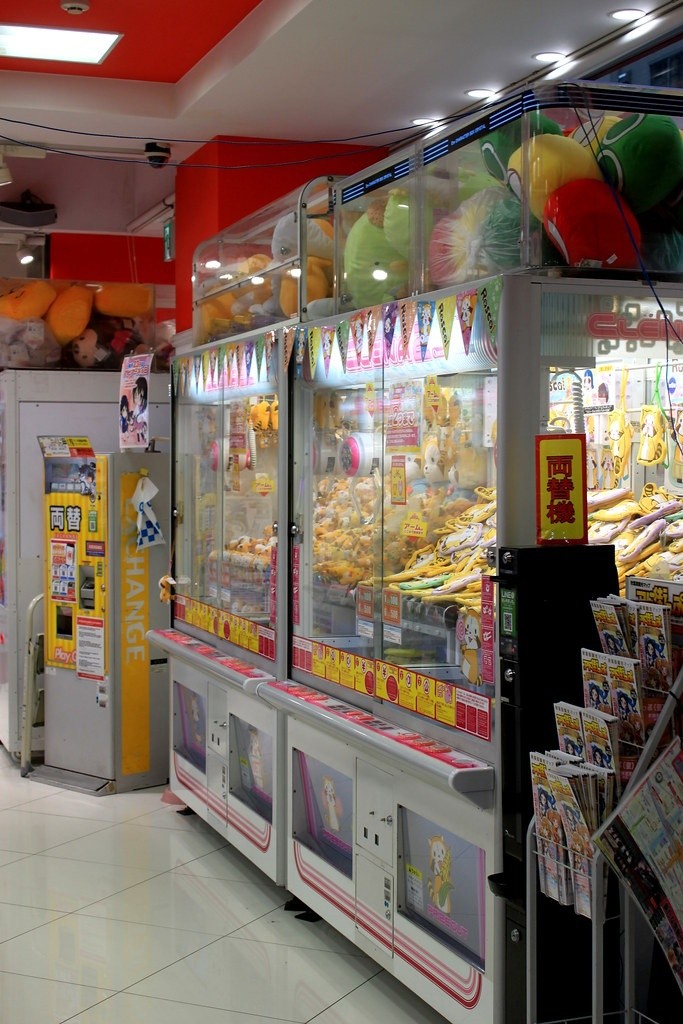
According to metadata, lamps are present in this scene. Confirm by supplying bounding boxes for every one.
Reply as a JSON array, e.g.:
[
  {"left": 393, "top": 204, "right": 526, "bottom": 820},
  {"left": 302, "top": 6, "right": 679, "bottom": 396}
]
[
  {"left": 15, "top": 234, "right": 33, "bottom": 264},
  {"left": 0, "top": 153, "right": 13, "bottom": 186}
]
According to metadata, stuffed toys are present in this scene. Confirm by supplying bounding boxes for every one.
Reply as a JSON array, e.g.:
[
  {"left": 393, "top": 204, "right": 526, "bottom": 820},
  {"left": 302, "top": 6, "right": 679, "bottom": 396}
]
[
  {"left": 250, "top": 401, "right": 279, "bottom": 432},
  {"left": 227, "top": 472, "right": 406, "bottom": 590},
  {"left": 397, "top": 439, "right": 487, "bottom": 508},
  {"left": 0, "top": 276, "right": 173, "bottom": 373},
  {"left": 197, "top": 108, "right": 683, "bottom": 358}
]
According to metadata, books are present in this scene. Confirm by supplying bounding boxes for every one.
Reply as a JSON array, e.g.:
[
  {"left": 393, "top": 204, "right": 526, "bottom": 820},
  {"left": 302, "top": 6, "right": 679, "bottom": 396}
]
[{"left": 530, "top": 577, "right": 683, "bottom": 994}]
